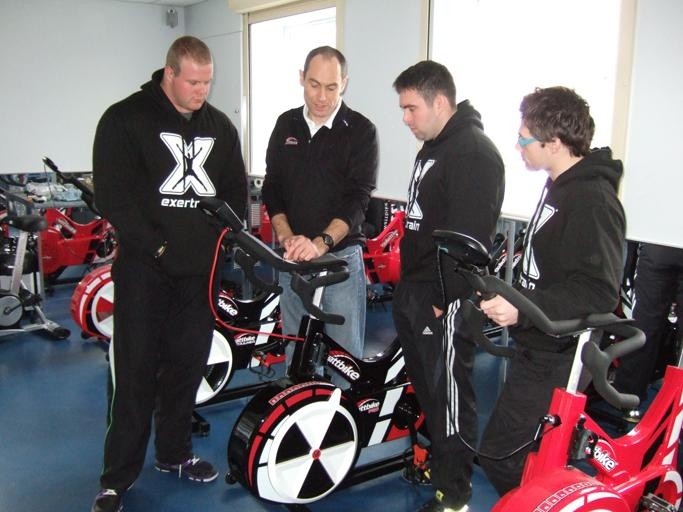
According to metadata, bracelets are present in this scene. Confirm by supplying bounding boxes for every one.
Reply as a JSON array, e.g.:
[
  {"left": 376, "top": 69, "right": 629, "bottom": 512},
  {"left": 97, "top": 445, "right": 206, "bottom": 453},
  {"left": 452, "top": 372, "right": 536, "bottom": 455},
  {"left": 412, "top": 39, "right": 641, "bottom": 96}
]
[{"left": 152, "top": 240, "right": 167, "bottom": 259}]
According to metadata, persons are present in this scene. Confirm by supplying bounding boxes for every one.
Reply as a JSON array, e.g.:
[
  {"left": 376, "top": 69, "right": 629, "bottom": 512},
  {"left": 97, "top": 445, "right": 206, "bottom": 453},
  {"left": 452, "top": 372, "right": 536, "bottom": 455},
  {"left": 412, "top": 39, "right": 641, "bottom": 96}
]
[
  {"left": 259, "top": 43, "right": 382, "bottom": 391},
  {"left": 473, "top": 86, "right": 631, "bottom": 500},
  {"left": 606, "top": 237, "right": 682, "bottom": 403},
  {"left": 89, "top": 34, "right": 251, "bottom": 512},
  {"left": 391, "top": 60, "right": 506, "bottom": 512}
]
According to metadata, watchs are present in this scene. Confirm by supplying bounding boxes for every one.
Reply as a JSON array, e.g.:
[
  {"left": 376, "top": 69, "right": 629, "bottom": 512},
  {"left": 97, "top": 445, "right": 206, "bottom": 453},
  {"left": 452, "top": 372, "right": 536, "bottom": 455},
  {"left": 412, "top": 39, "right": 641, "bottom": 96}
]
[{"left": 313, "top": 232, "right": 334, "bottom": 253}]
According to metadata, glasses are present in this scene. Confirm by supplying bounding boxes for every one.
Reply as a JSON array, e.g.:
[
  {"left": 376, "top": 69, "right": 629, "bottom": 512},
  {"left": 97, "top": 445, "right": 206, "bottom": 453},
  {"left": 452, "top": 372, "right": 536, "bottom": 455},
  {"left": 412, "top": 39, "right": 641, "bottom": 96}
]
[{"left": 518, "top": 137, "right": 537, "bottom": 146}]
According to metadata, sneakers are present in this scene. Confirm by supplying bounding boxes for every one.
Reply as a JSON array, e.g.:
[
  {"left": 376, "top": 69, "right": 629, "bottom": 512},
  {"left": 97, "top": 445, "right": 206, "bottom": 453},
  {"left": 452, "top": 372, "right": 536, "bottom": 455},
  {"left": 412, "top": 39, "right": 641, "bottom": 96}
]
[
  {"left": 401, "top": 461, "right": 432, "bottom": 486},
  {"left": 91, "top": 488, "right": 123, "bottom": 512},
  {"left": 151, "top": 455, "right": 219, "bottom": 482},
  {"left": 415, "top": 488, "right": 472, "bottom": 512}
]
